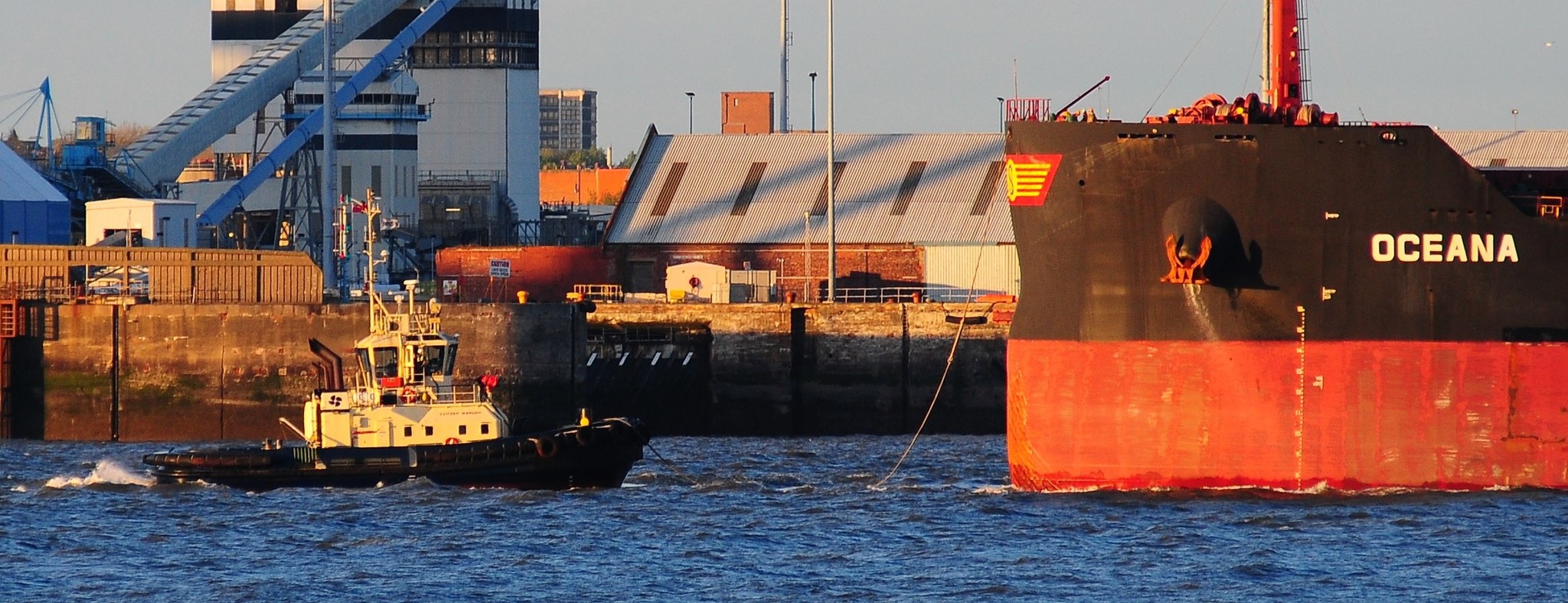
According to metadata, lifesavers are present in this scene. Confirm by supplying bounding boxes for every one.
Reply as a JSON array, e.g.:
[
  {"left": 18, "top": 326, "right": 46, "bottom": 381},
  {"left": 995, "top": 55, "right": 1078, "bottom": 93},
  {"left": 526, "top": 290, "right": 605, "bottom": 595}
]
[
  {"left": 403, "top": 389, "right": 417, "bottom": 403},
  {"left": 445, "top": 437, "right": 459, "bottom": 445},
  {"left": 608, "top": 421, "right": 625, "bottom": 437},
  {"left": 423, "top": 440, "right": 536, "bottom": 467},
  {"left": 575, "top": 426, "right": 595, "bottom": 446},
  {"left": 535, "top": 434, "right": 558, "bottom": 458}
]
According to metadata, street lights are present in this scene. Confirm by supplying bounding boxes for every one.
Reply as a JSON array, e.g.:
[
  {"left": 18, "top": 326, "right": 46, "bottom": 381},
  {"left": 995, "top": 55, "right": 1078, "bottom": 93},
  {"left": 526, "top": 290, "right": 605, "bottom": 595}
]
[
  {"left": 344, "top": 187, "right": 389, "bottom": 334},
  {"left": 685, "top": 92, "right": 695, "bottom": 134},
  {"left": 809, "top": 73, "right": 817, "bottom": 133},
  {"left": 997, "top": 97, "right": 1007, "bottom": 133}
]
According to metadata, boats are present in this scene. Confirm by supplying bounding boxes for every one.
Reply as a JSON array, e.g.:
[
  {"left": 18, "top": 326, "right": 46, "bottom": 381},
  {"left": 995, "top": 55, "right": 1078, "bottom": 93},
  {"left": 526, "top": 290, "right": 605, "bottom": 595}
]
[
  {"left": 143, "top": 279, "right": 651, "bottom": 489},
  {"left": 1006, "top": 122, "right": 1568, "bottom": 501}
]
